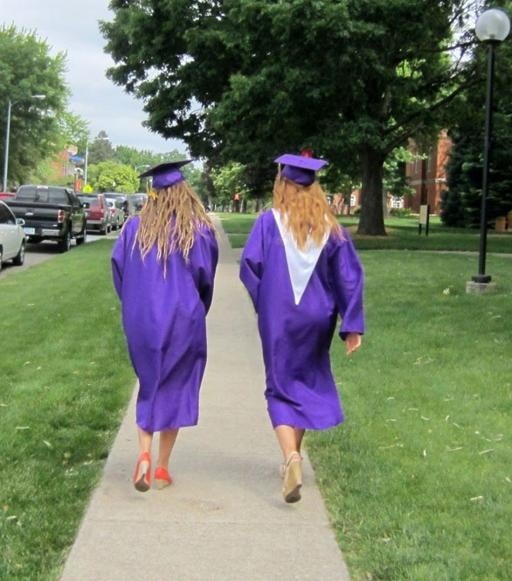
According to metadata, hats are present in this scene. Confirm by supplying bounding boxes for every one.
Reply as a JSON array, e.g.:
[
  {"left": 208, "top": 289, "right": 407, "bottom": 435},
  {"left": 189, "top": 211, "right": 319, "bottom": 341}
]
[
  {"left": 137, "top": 159, "right": 192, "bottom": 196},
  {"left": 274, "top": 154, "right": 327, "bottom": 186}
]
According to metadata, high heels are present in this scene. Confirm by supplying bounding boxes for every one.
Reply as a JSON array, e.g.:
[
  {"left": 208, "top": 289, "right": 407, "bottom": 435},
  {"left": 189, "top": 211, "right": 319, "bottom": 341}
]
[
  {"left": 134, "top": 453, "right": 151, "bottom": 492},
  {"left": 280, "top": 452, "right": 303, "bottom": 503},
  {"left": 154, "top": 467, "right": 172, "bottom": 489}
]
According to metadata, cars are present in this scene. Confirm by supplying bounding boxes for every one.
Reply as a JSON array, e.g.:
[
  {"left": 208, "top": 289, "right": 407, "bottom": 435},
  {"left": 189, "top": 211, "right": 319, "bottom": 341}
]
[{"left": 0, "top": 185, "right": 148, "bottom": 269}]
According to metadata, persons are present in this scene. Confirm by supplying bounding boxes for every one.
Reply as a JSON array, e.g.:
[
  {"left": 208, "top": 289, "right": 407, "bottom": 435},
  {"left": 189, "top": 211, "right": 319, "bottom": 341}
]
[
  {"left": 110, "top": 157, "right": 220, "bottom": 493},
  {"left": 237, "top": 153, "right": 367, "bottom": 504}
]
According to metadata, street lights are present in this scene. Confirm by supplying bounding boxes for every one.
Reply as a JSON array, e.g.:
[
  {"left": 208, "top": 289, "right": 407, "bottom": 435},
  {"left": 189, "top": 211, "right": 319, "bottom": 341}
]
[
  {"left": 84, "top": 138, "right": 109, "bottom": 184},
  {"left": 2, "top": 95, "right": 46, "bottom": 192},
  {"left": 135, "top": 165, "right": 150, "bottom": 172},
  {"left": 472, "top": 8, "right": 510, "bottom": 283}
]
